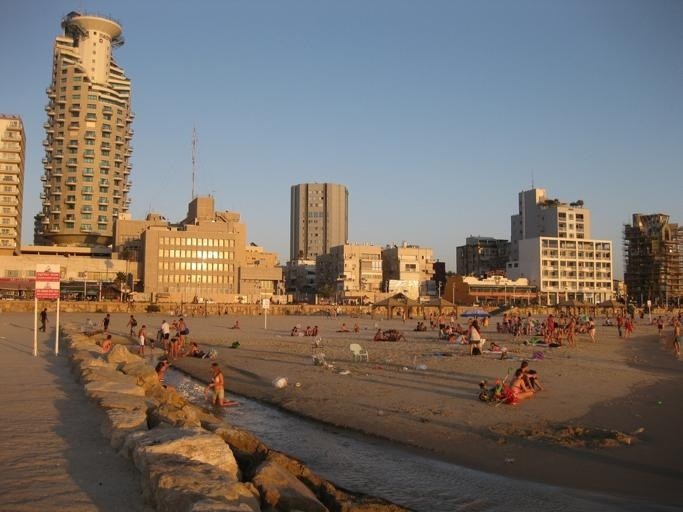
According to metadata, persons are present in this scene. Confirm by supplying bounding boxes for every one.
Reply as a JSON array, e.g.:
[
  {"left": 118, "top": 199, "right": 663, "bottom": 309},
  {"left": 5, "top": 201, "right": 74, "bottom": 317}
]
[
  {"left": 519, "top": 361, "right": 545, "bottom": 391},
  {"left": 209, "top": 362, "right": 226, "bottom": 407},
  {"left": 233, "top": 319, "right": 241, "bottom": 330},
  {"left": 374, "top": 304, "right": 683, "bottom": 359},
  {"left": 340, "top": 323, "right": 352, "bottom": 332},
  {"left": 39, "top": 307, "right": 51, "bottom": 332},
  {"left": 99, "top": 313, "right": 203, "bottom": 398},
  {"left": 510, "top": 369, "right": 533, "bottom": 399},
  {"left": 354, "top": 321, "right": 360, "bottom": 333},
  {"left": 289, "top": 326, "right": 320, "bottom": 337}
]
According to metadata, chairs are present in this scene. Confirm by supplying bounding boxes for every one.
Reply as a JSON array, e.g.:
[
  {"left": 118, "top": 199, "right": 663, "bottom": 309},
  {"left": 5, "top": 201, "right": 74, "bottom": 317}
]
[{"left": 349, "top": 343, "right": 368, "bottom": 362}]
